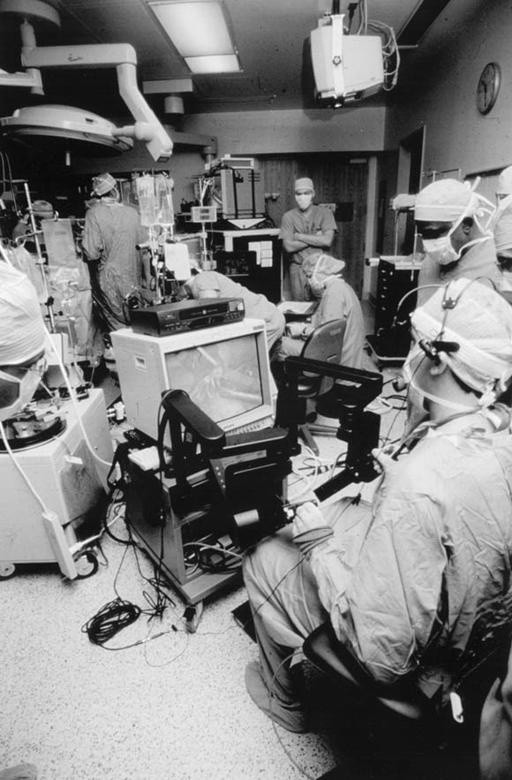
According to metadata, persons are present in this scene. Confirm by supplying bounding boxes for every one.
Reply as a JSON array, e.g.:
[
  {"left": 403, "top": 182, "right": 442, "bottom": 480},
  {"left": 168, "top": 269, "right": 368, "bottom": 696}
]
[
  {"left": 78, "top": 173, "right": 154, "bottom": 334},
  {"left": 481, "top": 202, "right": 511, "bottom": 300},
  {"left": 174, "top": 269, "right": 288, "bottom": 357},
  {"left": 491, "top": 164, "right": 511, "bottom": 202},
  {"left": 404, "top": 177, "right": 493, "bottom": 445},
  {"left": 270, "top": 252, "right": 373, "bottom": 387},
  {"left": 282, "top": 176, "right": 338, "bottom": 300},
  {"left": 246, "top": 276, "right": 511, "bottom": 731}
]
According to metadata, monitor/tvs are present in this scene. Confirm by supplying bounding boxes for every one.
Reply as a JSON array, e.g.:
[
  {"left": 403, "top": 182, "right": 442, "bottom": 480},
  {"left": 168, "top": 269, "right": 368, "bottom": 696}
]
[
  {"left": 309, "top": 25, "right": 385, "bottom": 108},
  {"left": 109, "top": 318, "right": 274, "bottom": 454}
]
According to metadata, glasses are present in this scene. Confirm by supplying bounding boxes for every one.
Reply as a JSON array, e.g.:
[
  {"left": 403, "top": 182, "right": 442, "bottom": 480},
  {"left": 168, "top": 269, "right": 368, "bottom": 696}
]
[{"left": 6, "top": 356, "right": 49, "bottom": 377}]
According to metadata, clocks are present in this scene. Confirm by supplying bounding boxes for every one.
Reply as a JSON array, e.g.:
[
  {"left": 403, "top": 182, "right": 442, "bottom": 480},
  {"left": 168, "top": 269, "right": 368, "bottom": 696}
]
[{"left": 475, "top": 59, "right": 501, "bottom": 114}]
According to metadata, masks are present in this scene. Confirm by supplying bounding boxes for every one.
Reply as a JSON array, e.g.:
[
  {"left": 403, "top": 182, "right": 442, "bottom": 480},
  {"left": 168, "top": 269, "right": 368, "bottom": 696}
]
[
  {"left": 306, "top": 273, "right": 325, "bottom": 292},
  {"left": 399, "top": 348, "right": 435, "bottom": 416},
  {"left": 115, "top": 188, "right": 121, "bottom": 202},
  {"left": 0, "top": 367, "right": 43, "bottom": 424},
  {"left": 294, "top": 193, "right": 313, "bottom": 212},
  {"left": 420, "top": 235, "right": 462, "bottom": 268}
]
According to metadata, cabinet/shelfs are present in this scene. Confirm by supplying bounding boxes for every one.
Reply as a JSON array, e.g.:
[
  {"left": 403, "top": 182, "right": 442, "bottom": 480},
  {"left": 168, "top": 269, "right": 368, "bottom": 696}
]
[
  {"left": 119, "top": 426, "right": 301, "bottom": 634},
  {"left": 0, "top": 387, "right": 122, "bottom": 585},
  {"left": 363, "top": 255, "right": 422, "bottom": 374}
]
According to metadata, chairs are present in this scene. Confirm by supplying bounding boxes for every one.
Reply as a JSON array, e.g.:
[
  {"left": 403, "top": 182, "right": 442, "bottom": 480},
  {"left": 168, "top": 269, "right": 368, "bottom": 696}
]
[
  {"left": 275, "top": 314, "right": 348, "bottom": 459},
  {"left": 296, "top": 580, "right": 512, "bottom": 779}
]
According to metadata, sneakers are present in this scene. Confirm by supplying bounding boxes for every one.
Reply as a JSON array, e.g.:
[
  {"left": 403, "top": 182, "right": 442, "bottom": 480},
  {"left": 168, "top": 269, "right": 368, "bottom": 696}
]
[{"left": 243, "top": 658, "right": 314, "bottom": 735}]
